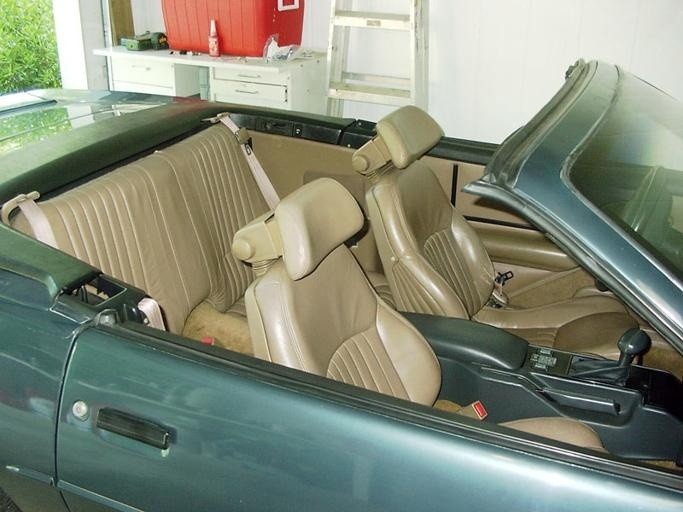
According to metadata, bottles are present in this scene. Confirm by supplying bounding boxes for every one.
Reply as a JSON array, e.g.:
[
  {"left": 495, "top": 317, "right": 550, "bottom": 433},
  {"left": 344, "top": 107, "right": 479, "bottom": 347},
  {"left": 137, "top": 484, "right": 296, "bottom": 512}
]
[{"left": 208, "top": 16, "right": 219, "bottom": 57}]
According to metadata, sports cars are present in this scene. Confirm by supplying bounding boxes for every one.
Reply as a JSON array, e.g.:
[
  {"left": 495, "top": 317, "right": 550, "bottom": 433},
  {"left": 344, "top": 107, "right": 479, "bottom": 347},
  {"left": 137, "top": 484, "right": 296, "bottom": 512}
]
[{"left": 0, "top": 54, "right": 683, "bottom": 511}]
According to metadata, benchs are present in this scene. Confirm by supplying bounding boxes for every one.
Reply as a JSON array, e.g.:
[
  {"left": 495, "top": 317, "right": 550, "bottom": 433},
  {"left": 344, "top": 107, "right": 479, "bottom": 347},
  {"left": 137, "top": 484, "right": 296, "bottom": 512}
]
[{"left": 14, "top": 118, "right": 397, "bottom": 371}]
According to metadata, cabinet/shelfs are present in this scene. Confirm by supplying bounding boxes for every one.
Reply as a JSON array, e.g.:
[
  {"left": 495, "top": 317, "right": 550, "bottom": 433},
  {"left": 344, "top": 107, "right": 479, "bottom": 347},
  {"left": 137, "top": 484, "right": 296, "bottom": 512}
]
[{"left": 93, "top": 41, "right": 327, "bottom": 118}]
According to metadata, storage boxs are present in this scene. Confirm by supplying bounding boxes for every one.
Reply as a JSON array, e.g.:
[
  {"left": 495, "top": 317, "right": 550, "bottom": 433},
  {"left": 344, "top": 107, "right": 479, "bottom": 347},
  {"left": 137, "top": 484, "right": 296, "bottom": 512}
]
[{"left": 161, "top": 0, "right": 304, "bottom": 58}]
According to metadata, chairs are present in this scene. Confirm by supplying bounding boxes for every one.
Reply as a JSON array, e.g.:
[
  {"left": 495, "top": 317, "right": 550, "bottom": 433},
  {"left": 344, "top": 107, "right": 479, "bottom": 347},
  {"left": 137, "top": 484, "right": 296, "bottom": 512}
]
[
  {"left": 351, "top": 101, "right": 644, "bottom": 372},
  {"left": 229, "top": 173, "right": 615, "bottom": 459}
]
